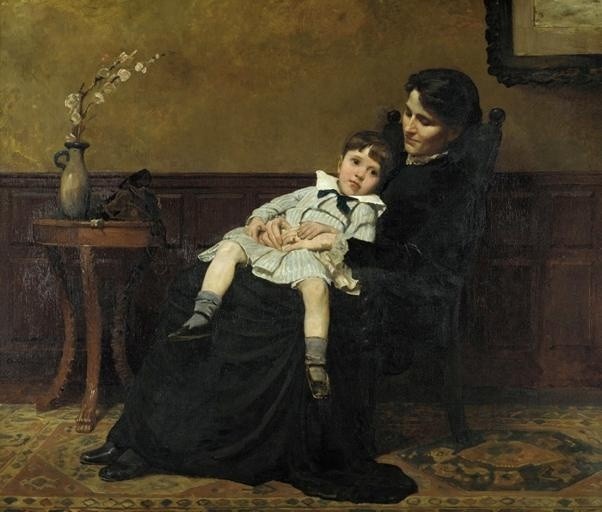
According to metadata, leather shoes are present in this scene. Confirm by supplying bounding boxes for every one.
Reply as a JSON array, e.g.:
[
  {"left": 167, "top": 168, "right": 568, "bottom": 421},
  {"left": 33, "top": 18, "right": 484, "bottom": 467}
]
[
  {"left": 99, "top": 447, "right": 146, "bottom": 481},
  {"left": 79, "top": 442, "right": 122, "bottom": 464}
]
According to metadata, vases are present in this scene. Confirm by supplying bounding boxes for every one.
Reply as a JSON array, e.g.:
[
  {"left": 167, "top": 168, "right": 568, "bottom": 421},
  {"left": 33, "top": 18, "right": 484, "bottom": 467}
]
[{"left": 53, "top": 142, "right": 92, "bottom": 218}]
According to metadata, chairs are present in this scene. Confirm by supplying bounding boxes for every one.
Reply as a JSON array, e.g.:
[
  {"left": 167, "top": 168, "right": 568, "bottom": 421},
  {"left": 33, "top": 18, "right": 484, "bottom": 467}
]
[{"left": 347, "top": 106, "right": 507, "bottom": 451}]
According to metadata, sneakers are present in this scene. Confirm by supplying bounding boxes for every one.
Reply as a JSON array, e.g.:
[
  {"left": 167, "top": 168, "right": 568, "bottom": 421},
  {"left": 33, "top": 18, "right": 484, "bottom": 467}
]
[
  {"left": 304, "top": 365, "right": 331, "bottom": 400},
  {"left": 167, "top": 322, "right": 210, "bottom": 341}
]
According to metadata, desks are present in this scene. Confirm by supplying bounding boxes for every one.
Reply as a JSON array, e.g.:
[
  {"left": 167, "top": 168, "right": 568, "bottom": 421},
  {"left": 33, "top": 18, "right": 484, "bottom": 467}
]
[{"left": 31, "top": 218, "right": 163, "bottom": 435}]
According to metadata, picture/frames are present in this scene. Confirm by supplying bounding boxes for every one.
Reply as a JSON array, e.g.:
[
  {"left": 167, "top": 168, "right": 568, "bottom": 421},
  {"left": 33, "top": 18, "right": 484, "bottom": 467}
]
[{"left": 483, "top": 0, "right": 602, "bottom": 88}]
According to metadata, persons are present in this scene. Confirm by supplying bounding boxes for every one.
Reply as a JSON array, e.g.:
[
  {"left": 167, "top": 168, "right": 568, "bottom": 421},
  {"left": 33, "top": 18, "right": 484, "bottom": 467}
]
[
  {"left": 166, "top": 132, "right": 401, "bottom": 401},
  {"left": 78, "top": 67, "right": 488, "bottom": 504}
]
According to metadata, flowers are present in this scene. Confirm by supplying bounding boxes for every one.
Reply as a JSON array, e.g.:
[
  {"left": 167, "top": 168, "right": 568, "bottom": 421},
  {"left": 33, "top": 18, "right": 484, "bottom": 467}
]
[{"left": 61, "top": 47, "right": 175, "bottom": 141}]
